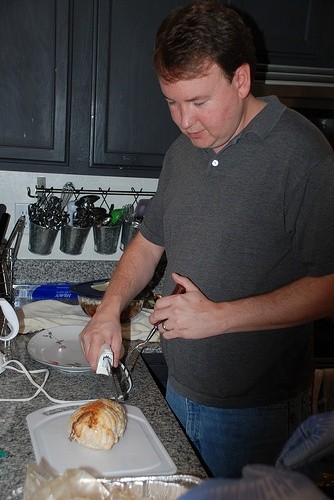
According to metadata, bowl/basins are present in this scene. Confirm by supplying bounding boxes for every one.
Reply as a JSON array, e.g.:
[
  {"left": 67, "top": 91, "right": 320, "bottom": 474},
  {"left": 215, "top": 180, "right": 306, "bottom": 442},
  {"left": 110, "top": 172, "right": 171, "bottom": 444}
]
[
  {"left": 6, "top": 476, "right": 204, "bottom": 499},
  {"left": 77, "top": 295, "right": 145, "bottom": 323}
]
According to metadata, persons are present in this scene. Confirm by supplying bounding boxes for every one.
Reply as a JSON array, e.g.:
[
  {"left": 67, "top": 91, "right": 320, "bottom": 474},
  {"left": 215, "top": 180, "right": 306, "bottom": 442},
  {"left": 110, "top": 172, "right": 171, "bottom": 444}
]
[
  {"left": 178, "top": 410, "right": 334, "bottom": 500},
  {"left": 80, "top": 1, "right": 334, "bottom": 478}
]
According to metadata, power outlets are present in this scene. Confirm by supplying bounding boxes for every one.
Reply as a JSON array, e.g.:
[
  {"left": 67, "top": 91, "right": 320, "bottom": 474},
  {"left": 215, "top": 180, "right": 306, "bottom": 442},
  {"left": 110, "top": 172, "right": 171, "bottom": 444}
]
[{"left": 15, "top": 204, "right": 30, "bottom": 234}]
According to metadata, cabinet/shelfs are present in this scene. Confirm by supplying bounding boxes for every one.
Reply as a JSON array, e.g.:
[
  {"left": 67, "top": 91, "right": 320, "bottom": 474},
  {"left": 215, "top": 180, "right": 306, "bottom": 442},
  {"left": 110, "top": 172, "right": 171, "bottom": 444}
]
[{"left": 0, "top": 0, "right": 334, "bottom": 179}]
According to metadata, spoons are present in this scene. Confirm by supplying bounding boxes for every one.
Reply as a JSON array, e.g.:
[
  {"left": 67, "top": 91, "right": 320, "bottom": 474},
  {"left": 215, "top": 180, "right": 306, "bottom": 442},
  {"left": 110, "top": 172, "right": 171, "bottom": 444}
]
[{"left": 28, "top": 196, "right": 143, "bottom": 227}]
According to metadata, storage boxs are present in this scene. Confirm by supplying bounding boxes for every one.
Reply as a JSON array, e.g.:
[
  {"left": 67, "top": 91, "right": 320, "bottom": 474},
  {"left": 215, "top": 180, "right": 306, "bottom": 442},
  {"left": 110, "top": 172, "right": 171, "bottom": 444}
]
[{"left": 13, "top": 283, "right": 80, "bottom": 299}]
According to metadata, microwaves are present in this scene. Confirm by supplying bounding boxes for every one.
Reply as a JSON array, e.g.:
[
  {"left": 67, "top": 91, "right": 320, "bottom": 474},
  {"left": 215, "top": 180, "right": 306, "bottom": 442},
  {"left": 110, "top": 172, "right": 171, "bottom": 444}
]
[{"left": 260, "top": 71, "right": 333, "bottom": 153}]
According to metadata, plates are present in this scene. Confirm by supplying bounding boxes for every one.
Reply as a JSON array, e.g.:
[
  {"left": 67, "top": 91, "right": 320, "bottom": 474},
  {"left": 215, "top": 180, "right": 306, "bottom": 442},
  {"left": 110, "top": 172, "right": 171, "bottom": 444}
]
[{"left": 27, "top": 326, "right": 125, "bottom": 373}]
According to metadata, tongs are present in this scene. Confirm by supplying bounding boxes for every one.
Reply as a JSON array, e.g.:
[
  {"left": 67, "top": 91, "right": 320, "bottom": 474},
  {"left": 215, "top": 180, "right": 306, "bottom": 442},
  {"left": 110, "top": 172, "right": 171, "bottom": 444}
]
[{"left": 0, "top": 216, "right": 24, "bottom": 266}]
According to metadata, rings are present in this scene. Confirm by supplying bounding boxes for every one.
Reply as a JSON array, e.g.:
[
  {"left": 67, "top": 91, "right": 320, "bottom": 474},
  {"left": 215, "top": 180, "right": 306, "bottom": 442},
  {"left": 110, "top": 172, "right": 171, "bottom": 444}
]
[{"left": 162, "top": 320, "right": 170, "bottom": 332}]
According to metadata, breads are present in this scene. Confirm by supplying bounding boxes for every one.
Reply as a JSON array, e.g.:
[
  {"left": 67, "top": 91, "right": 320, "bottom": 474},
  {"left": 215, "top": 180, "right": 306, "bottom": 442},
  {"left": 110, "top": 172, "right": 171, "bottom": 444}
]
[{"left": 68, "top": 398, "right": 128, "bottom": 449}]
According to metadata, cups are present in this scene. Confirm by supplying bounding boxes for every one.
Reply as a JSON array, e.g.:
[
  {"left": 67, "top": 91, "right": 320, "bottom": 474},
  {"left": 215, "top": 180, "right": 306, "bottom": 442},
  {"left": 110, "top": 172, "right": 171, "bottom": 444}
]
[
  {"left": 28, "top": 222, "right": 58, "bottom": 255},
  {"left": 120, "top": 223, "right": 142, "bottom": 251},
  {"left": 93, "top": 223, "right": 122, "bottom": 254},
  {"left": 60, "top": 223, "right": 90, "bottom": 255}
]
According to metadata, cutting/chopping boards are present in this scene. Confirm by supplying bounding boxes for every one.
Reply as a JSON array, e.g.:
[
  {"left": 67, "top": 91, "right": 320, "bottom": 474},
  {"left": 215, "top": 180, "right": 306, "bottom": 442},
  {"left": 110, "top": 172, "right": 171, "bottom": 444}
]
[{"left": 25, "top": 400, "right": 177, "bottom": 481}]
